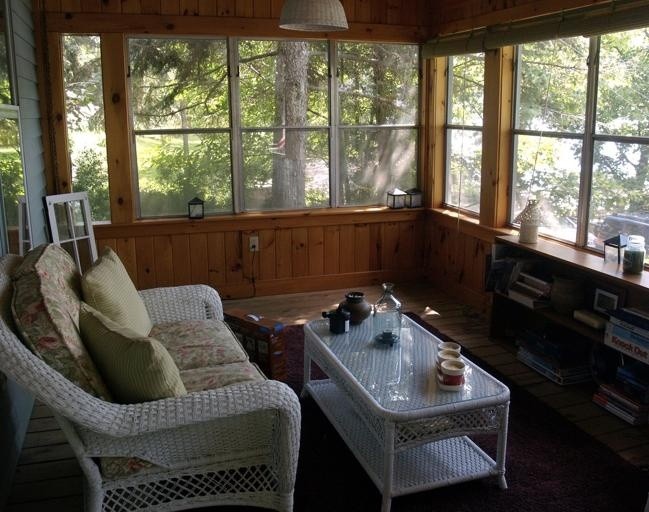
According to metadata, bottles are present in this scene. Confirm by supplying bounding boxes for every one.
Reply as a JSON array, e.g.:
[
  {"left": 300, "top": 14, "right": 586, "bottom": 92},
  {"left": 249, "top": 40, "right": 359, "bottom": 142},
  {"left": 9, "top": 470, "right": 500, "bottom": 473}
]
[
  {"left": 329, "top": 307, "right": 351, "bottom": 334},
  {"left": 622, "top": 234, "right": 645, "bottom": 274},
  {"left": 372, "top": 281, "right": 402, "bottom": 346}
]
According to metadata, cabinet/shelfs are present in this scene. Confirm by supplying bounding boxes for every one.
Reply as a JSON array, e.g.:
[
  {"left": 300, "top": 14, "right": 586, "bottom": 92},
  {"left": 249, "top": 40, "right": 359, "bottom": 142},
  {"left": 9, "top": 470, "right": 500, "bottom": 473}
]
[{"left": 487, "top": 231, "right": 649, "bottom": 432}]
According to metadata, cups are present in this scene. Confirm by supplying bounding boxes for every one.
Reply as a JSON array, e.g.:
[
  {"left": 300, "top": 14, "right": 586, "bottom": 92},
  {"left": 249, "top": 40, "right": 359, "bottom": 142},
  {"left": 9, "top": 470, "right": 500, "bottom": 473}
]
[{"left": 435, "top": 340, "right": 466, "bottom": 391}]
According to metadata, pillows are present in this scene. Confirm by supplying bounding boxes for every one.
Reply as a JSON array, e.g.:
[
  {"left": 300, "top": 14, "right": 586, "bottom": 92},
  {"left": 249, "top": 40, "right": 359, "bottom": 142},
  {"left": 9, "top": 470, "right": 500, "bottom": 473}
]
[
  {"left": 75, "top": 243, "right": 155, "bottom": 339},
  {"left": 78, "top": 295, "right": 185, "bottom": 401}
]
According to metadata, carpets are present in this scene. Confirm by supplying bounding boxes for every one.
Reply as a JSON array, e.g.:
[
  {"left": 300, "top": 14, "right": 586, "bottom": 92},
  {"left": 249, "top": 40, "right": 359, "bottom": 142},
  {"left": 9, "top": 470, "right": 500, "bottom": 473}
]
[{"left": 206, "top": 306, "right": 648, "bottom": 512}]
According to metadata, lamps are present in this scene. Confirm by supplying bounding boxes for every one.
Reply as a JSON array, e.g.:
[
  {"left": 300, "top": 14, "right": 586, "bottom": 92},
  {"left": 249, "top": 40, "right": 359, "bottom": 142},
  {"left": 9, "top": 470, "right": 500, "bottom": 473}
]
[
  {"left": 602, "top": 234, "right": 627, "bottom": 265},
  {"left": 189, "top": 197, "right": 204, "bottom": 218},
  {"left": 405, "top": 187, "right": 424, "bottom": 208},
  {"left": 386, "top": 187, "right": 407, "bottom": 208},
  {"left": 275, "top": 0, "right": 352, "bottom": 35}
]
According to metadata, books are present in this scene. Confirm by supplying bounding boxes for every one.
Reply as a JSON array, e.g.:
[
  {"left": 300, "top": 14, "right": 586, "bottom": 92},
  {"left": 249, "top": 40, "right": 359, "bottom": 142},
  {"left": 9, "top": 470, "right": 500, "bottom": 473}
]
[{"left": 482, "top": 241, "right": 649, "bottom": 428}]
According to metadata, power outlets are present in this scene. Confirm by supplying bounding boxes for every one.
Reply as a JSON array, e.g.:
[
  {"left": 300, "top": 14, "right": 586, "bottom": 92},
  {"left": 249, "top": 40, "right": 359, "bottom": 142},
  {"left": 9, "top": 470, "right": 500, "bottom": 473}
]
[{"left": 247, "top": 235, "right": 260, "bottom": 253}]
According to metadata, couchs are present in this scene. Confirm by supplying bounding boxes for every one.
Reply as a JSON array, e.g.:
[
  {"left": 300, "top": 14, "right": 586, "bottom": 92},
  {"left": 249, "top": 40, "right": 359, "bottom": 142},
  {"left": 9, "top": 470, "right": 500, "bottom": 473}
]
[{"left": 0, "top": 239, "right": 308, "bottom": 512}]
